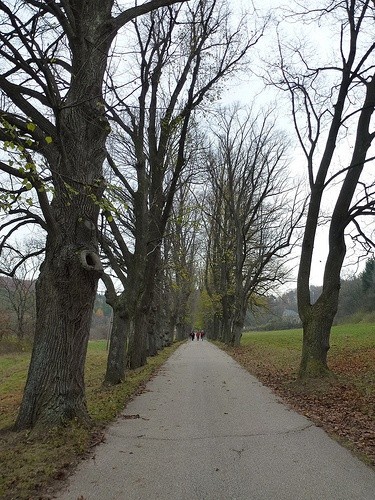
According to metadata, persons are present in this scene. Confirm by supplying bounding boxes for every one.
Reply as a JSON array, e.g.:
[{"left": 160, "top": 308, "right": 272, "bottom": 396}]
[
  {"left": 201, "top": 330, "right": 205, "bottom": 341},
  {"left": 191, "top": 331, "right": 195, "bottom": 341},
  {"left": 196, "top": 330, "right": 200, "bottom": 341}
]
